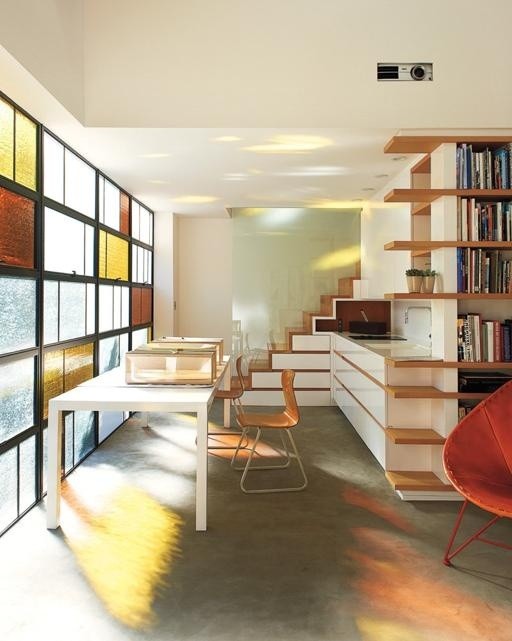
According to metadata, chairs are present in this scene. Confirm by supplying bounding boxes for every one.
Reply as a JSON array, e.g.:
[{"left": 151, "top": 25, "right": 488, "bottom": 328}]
[
  {"left": 195, "top": 356, "right": 308, "bottom": 495},
  {"left": 440, "top": 378, "right": 511, "bottom": 566}
]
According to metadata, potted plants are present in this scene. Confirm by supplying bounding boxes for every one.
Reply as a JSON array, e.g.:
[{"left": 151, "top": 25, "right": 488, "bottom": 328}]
[{"left": 407, "top": 264, "right": 437, "bottom": 294}]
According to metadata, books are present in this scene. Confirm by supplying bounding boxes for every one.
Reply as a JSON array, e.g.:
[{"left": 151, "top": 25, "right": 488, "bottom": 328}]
[
  {"left": 459, "top": 402, "right": 472, "bottom": 423},
  {"left": 457, "top": 143, "right": 512, "bottom": 294},
  {"left": 458, "top": 371, "right": 512, "bottom": 393},
  {"left": 457, "top": 312, "right": 512, "bottom": 362}
]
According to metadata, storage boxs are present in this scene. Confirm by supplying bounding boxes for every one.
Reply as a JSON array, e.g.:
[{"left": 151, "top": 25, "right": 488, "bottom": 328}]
[{"left": 125, "top": 336, "right": 224, "bottom": 385}]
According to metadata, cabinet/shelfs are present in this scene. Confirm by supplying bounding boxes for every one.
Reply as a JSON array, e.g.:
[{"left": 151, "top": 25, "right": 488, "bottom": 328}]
[{"left": 382, "top": 134, "right": 512, "bottom": 504}]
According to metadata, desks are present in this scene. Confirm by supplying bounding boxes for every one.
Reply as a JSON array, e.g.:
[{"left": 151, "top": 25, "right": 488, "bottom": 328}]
[{"left": 44, "top": 354, "right": 232, "bottom": 533}]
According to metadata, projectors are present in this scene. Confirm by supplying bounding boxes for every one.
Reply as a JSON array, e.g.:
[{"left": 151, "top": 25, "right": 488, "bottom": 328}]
[{"left": 378, "top": 64, "right": 432, "bottom": 80}]
